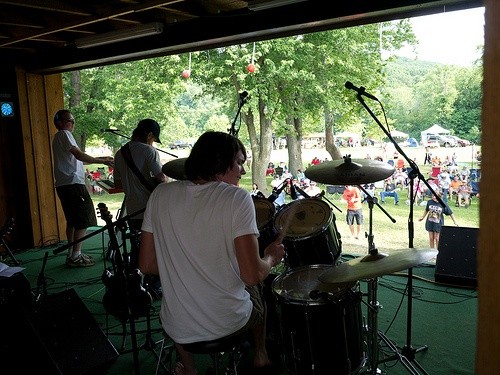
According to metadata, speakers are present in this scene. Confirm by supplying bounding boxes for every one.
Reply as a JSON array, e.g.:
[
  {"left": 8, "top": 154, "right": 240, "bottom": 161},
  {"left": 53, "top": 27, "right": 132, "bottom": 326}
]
[
  {"left": 3, "top": 290, "right": 119, "bottom": 375},
  {"left": 433, "top": 225, "right": 480, "bottom": 291}
]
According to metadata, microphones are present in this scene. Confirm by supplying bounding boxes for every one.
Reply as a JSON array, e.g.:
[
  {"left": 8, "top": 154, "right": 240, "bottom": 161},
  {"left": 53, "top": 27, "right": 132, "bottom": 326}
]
[
  {"left": 290, "top": 184, "right": 297, "bottom": 199},
  {"left": 100, "top": 128, "right": 120, "bottom": 132},
  {"left": 345, "top": 82, "right": 381, "bottom": 104},
  {"left": 268, "top": 190, "right": 282, "bottom": 202},
  {"left": 238, "top": 92, "right": 249, "bottom": 103}
]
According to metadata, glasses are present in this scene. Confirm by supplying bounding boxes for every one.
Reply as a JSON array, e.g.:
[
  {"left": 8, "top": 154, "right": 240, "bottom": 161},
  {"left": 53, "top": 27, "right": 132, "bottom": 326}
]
[{"left": 60, "top": 119, "right": 75, "bottom": 124}]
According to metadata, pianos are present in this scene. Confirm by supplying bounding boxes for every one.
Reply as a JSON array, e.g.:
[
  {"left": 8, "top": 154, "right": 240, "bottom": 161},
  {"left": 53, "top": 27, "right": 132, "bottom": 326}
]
[{"left": 96, "top": 179, "right": 128, "bottom": 258}]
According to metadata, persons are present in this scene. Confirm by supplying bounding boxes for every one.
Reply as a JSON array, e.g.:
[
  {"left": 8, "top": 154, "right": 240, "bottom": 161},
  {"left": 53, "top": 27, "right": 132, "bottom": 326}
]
[
  {"left": 53, "top": 109, "right": 114, "bottom": 266},
  {"left": 418, "top": 190, "right": 459, "bottom": 249},
  {"left": 246, "top": 134, "right": 480, "bottom": 205},
  {"left": 112, "top": 117, "right": 166, "bottom": 301},
  {"left": 343, "top": 185, "right": 364, "bottom": 239},
  {"left": 138, "top": 129, "right": 285, "bottom": 375}
]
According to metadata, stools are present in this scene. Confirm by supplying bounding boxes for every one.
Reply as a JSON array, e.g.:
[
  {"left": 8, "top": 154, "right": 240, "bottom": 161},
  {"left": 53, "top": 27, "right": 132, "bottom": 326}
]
[{"left": 181, "top": 330, "right": 248, "bottom": 375}]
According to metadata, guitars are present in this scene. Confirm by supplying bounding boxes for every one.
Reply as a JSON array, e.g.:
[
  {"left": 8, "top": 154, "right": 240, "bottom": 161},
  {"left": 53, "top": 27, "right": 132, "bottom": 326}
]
[{"left": 96, "top": 203, "right": 153, "bottom": 321}]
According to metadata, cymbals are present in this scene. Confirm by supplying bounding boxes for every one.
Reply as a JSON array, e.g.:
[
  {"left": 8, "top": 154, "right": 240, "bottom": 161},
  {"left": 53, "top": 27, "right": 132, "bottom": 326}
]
[
  {"left": 319, "top": 248, "right": 440, "bottom": 284},
  {"left": 305, "top": 158, "right": 394, "bottom": 185}
]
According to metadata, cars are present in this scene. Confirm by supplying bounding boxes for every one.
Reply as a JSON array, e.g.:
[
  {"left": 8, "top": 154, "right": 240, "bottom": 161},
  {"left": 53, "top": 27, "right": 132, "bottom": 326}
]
[
  {"left": 427, "top": 135, "right": 457, "bottom": 148},
  {"left": 446, "top": 135, "right": 470, "bottom": 147}
]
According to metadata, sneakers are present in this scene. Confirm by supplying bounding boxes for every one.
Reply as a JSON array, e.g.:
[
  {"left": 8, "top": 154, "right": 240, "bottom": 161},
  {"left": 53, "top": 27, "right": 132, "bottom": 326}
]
[{"left": 66, "top": 253, "right": 96, "bottom": 268}]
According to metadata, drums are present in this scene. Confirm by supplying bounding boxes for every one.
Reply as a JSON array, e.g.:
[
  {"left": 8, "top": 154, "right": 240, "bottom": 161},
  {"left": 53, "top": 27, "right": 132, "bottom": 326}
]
[
  {"left": 275, "top": 199, "right": 342, "bottom": 263},
  {"left": 272, "top": 264, "right": 365, "bottom": 375},
  {"left": 252, "top": 198, "right": 274, "bottom": 257}
]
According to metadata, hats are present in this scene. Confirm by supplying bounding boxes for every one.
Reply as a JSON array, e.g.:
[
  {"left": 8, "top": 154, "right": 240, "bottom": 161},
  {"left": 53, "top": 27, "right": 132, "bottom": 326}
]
[{"left": 137, "top": 119, "right": 163, "bottom": 145}]
